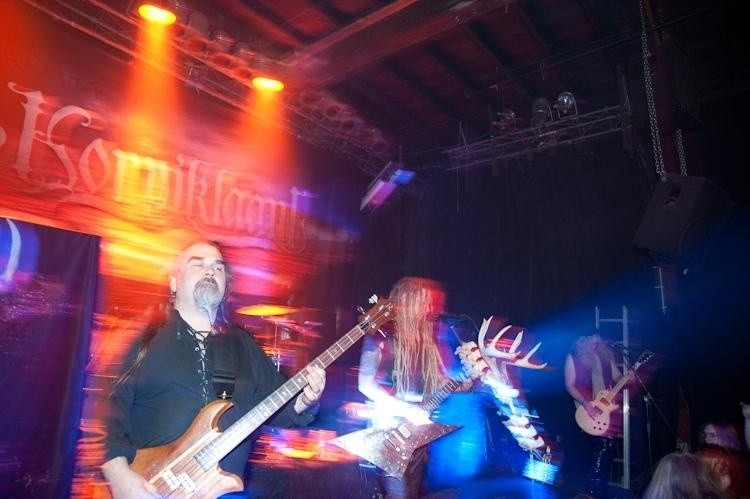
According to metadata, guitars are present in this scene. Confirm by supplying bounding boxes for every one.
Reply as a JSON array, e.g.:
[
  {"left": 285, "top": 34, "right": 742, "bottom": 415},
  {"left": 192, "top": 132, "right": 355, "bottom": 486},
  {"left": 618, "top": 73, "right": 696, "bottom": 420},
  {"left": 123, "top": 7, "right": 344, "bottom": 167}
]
[
  {"left": 91, "top": 294, "right": 398, "bottom": 499},
  {"left": 329, "top": 363, "right": 471, "bottom": 499},
  {"left": 575, "top": 348, "right": 655, "bottom": 436}
]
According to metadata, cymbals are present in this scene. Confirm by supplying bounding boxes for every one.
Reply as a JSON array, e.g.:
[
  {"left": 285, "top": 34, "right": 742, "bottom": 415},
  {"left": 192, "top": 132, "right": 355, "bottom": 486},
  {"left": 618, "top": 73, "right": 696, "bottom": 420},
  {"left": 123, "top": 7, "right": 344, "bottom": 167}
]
[
  {"left": 279, "top": 341, "right": 313, "bottom": 347},
  {"left": 233, "top": 304, "right": 298, "bottom": 316},
  {"left": 252, "top": 333, "right": 279, "bottom": 339}
]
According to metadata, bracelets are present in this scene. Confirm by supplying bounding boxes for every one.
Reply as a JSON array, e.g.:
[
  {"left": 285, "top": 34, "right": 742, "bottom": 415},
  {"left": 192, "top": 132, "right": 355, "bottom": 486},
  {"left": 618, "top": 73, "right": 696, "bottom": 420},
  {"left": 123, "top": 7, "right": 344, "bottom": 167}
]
[
  {"left": 582, "top": 399, "right": 585, "bottom": 405},
  {"left": 301, "top": 394, "right": 318, "bottom": 408},
  {"left": 460, "top": 385, "right": 464, "bottom": 393}
]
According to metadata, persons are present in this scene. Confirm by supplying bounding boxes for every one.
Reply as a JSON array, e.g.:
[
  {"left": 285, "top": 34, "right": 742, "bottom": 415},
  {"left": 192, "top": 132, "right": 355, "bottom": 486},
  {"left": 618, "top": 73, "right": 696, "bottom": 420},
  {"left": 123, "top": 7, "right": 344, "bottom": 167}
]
[
  {"left": 641, "top": 395, "right": 750, "bottom": 499},
  {"left": 102, "top": 240, "right": 326, "bottom": 499},
  {"left": 359, "top": 276, "right": 474, "bottom": 499},
  {"left": 565, "top": 327, "right": 636, "bottom": 499}
]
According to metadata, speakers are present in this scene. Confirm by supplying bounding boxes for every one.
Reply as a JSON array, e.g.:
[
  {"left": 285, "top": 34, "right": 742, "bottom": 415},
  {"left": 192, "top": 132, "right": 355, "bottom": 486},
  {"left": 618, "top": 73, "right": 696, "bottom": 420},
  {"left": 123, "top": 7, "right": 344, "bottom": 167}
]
[{"left": 1, "top": 216, "right": 101, "bottom": 499}]
